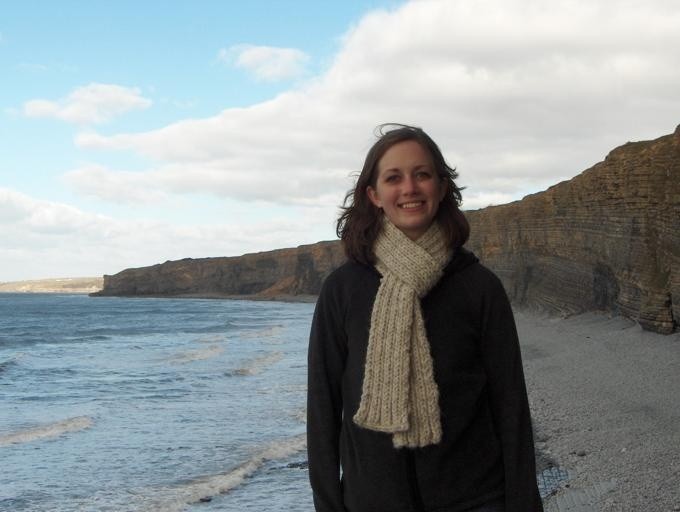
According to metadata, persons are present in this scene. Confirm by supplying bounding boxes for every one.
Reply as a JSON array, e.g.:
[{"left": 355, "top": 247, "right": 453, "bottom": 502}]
[{"left": 303, "top": 122, "right": 543, "bottom": 510}]
